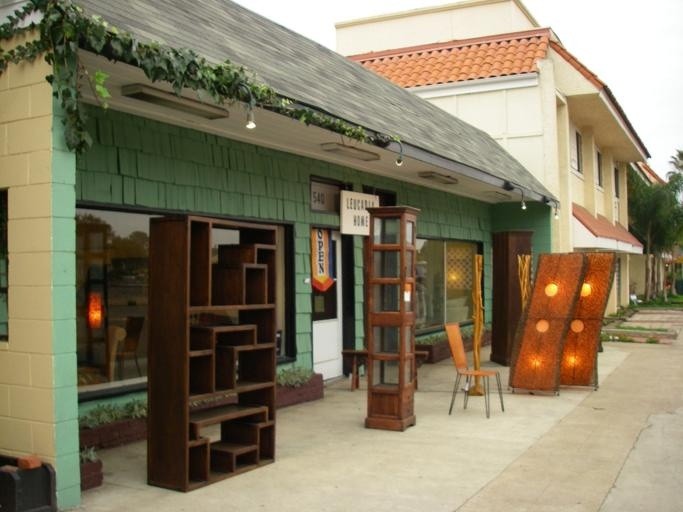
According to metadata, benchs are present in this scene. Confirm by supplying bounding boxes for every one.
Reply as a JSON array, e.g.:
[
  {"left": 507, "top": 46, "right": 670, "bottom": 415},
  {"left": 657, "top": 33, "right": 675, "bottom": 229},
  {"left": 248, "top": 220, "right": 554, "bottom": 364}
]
[{"left": 341, "top": 349, "right": 429, "bottom": 392}]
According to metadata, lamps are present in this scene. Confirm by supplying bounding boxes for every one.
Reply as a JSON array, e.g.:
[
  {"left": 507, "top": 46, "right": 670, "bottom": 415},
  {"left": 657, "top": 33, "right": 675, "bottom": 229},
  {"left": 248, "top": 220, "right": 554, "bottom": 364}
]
[
  {"left": 372, "top": 135, "right": 403, "bottom": 166},
  {"left": 503, "top": 180, "right": 526, "bottom": 210},
  {"left": 237, "top": 81, "right": 256, "bottom": 129}
]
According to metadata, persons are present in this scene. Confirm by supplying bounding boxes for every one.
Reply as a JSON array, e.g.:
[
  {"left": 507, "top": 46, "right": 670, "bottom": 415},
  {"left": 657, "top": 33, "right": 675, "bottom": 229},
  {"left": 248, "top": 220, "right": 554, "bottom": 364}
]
[{"left": 630, "top": 278, "right": 671, "bottom": 306}]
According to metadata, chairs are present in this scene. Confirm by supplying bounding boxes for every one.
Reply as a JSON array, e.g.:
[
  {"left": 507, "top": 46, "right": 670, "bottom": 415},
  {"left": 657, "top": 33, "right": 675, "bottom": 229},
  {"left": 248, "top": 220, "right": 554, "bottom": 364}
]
[{"left": 442, "top": 321, "right": 504, "bottom": 418}]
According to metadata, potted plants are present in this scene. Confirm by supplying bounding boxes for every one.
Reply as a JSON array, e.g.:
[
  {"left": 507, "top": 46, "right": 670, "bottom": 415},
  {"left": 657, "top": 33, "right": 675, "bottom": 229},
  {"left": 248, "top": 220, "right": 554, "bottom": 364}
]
[
  {"left": 414, "top": 326, "right": 492, "bottom": 364},
  {"left": 77, "top": 397, "right": 147, "bottom": 491},
  {"left": 275, "top": 361, "right": 323, "bottom": 409}
]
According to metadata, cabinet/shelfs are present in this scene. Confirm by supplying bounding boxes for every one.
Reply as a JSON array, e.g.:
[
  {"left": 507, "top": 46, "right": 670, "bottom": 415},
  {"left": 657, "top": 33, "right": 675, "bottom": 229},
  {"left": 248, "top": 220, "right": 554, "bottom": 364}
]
[
  {"left": 363, "top": 204, "right": 421, "bottom": 432},
  {"left": 146, "top": 213, "right": 278, "bottom": 495}
]
